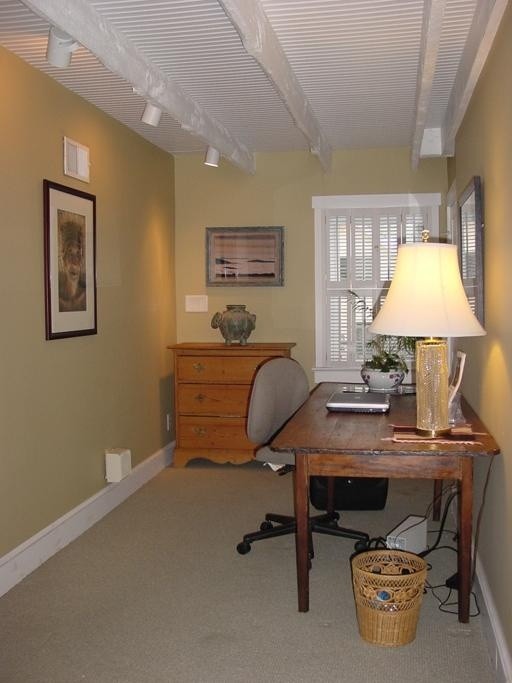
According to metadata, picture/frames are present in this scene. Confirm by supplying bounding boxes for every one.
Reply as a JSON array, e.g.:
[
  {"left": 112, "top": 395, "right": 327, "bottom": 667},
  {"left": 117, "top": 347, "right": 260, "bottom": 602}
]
[
  {"left": 204, "top": 226, "right": 286, "bottom": 289},
  {"left": 43, "top": 178, "right": 97, "bottom": 340},
  {"left": 455, "top": 173, "right": 486, "bottom": 333}
]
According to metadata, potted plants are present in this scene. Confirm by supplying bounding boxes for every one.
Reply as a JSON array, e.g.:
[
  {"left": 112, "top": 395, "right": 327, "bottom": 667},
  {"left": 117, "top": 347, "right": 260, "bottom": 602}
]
[{"left": 348, "top": 290, "right": 418, "bottom": 395}]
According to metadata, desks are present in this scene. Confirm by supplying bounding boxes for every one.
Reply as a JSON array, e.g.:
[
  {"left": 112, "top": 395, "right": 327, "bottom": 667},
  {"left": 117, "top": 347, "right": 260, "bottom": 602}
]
[{"left": 267, "top": 380, "right": 501, "bottom": 624}]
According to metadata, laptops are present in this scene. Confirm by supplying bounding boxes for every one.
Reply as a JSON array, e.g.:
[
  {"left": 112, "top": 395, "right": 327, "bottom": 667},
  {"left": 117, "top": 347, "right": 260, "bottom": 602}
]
[{"left": 326, "top": 390, "right": 391, "bottom": 413}]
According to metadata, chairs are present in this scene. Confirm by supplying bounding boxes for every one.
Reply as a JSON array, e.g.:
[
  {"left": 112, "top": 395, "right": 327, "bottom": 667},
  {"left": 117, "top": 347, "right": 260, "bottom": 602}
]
[{"left": 237, "top": 354, "right": 370, "bottom": 569}]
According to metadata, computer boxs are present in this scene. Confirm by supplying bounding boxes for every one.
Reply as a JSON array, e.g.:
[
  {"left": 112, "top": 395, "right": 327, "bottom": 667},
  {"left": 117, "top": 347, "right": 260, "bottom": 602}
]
[{"left": 387, "top": 514, "right": 428, "bottom": 554}]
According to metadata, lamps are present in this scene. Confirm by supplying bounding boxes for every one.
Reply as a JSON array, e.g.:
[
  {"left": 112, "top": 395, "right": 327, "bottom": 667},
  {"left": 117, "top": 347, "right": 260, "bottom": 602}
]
[
  {"left": 45, "top": 25, "right": 75, "bottom": 66},
  {"left": 204, "top": 145, "right": 220, "bottom": 167},
  {"left": 140, "top": 100, "right": 162, "bottom": 127},
  {"left": 367, "top": 230, "right": 487, "bottom": 439}
]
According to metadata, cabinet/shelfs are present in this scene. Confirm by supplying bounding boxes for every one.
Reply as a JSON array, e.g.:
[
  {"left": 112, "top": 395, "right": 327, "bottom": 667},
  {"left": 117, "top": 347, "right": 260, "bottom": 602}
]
[{"left": 167, "top": 341, "right": 297, "bottom": 469}]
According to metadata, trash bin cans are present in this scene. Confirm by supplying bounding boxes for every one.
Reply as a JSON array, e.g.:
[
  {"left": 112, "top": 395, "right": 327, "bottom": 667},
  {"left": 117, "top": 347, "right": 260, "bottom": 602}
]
[{"left": 351, "top": 549, "right": 427, "bottom": 647}]
